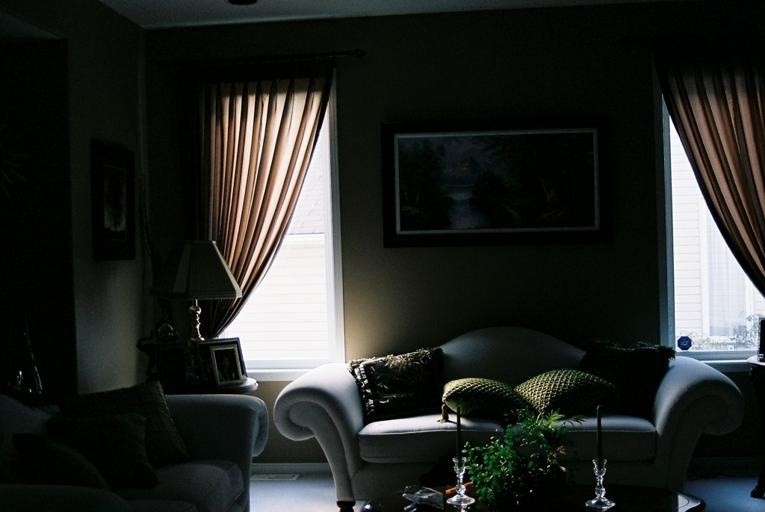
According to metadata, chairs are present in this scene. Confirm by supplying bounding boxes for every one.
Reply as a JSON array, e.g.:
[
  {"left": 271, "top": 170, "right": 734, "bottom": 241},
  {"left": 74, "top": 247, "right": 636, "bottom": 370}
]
[{"left": 0, "top": 361, "right": 271, "bottom": 512}]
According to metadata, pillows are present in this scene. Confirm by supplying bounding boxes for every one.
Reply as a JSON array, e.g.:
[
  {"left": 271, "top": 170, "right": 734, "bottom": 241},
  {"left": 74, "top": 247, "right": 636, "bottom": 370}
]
[{"left": 12, "top": 373, "right": 192, "bottom": 500}]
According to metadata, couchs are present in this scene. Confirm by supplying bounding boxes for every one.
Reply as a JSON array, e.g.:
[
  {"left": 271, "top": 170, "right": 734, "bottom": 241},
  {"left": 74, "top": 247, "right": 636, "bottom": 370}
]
[{"left": 273, "top": 321, "right": 748, "bottom": 512}]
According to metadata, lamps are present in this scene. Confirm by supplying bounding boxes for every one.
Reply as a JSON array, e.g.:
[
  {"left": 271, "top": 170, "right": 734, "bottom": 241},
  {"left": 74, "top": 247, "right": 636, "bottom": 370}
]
[{"left": 151, "top": 237, "right": 243, "bottom": 343}]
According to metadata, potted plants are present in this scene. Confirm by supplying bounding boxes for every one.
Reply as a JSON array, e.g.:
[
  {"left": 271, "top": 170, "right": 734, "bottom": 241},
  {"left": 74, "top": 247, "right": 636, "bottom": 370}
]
[{"left": 463, "top": 406, "right": 586, "bottom": 506}]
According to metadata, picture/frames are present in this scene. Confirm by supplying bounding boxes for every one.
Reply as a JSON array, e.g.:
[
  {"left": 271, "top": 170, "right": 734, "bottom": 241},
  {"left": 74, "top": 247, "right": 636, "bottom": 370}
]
[
  {"left": 206, "top": 344, "right": 243, "bottom": 389},
  {"left": 88, "top": 134, "right": 136, "bottom": 262},
  {"left": 374, "top": 113, "right": 614, "bottom": 251}
]
